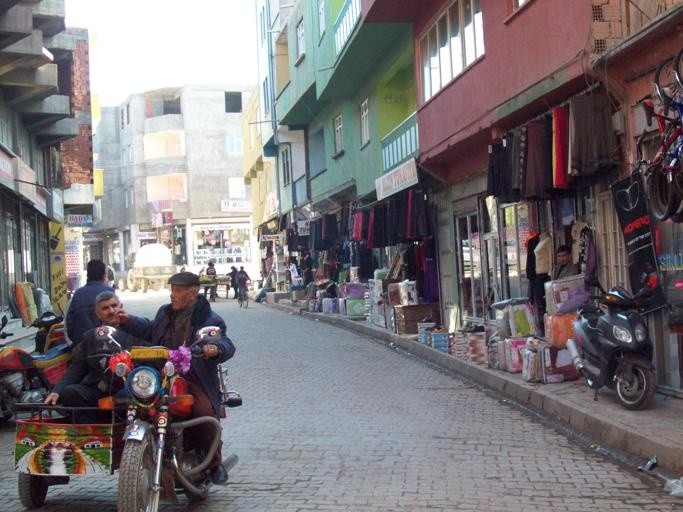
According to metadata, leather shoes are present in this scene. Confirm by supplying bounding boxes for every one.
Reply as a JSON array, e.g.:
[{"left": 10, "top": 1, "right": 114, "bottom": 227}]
[
  {"left": 458, "top": 325, "right": 484, "bottom": 332},
  {"left": 211, "top": 465, "right": 227, "bottom": 483}
]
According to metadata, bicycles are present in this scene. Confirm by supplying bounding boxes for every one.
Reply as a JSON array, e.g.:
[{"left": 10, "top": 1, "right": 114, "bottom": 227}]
[
  {"left": 237, "top": 278, "right": 251, "bottom": 309},
  {"left": 631, "top": 46, "right": 683, "bottom": 224}
]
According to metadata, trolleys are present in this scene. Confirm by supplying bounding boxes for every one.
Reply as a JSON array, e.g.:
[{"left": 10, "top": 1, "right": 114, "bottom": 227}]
[{"left": 66, "top": 274, "right": 82, "bottom": 300}]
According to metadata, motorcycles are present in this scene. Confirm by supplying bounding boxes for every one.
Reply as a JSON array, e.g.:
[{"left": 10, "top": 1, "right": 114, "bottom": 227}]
[
  {"left": 13, "top": 324, "right": 242, "bottom": 512},
  {"left": 0, "top": 302, "right": 72, "bottom": 425},
  {"left": 0, "top": 315, "right": 14, "bottom": 348},
  {"left": 564, "top": 271, "right": 666, "bottom": 412}
]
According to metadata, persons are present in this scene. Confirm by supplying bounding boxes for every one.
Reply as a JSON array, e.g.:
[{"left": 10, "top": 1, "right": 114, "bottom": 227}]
[
  {"left": 45, "top": 290, "right": 149, "bottom": 425},
  {"left": 552, "top": 245, "right": 577, "bottom": 280},
  {"left": 67, "top": 259, "right": 114, "bottom": 345},
  {"left": 116, "top": 271, "right": 236, "bottom": 484},
  {"left": 202, "top": 261, "right": 251, "bottom": 307}
]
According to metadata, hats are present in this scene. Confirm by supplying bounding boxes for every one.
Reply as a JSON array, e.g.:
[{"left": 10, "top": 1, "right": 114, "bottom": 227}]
[{"left": 169, "top": 272, "right": 200, "bottom": 285}]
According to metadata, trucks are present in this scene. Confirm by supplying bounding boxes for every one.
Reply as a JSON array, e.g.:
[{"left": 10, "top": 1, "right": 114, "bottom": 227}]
[{"left": 115, "top": 243, "right": 179, "bottom": 293}]
